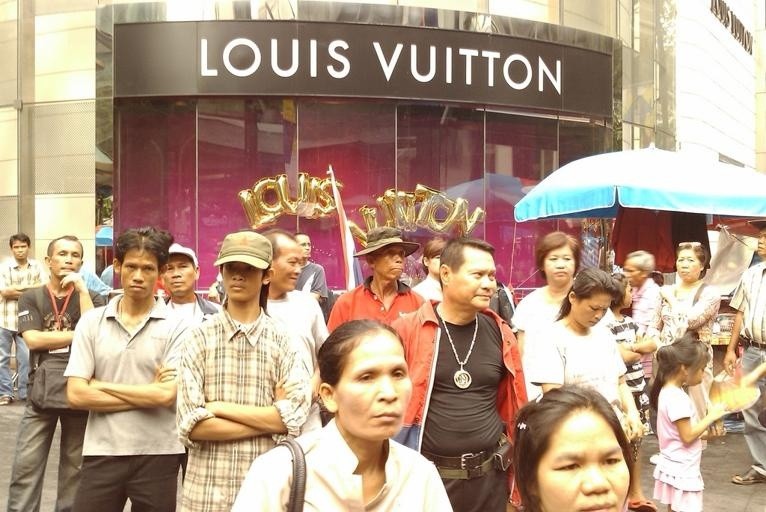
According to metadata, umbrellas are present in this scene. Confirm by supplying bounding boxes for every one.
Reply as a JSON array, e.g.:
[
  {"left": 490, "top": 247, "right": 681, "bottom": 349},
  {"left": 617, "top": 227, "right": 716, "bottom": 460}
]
[
  {"left": 408, "top": 175, "right": 539, "bottom": 226},
  {"left": 515, "top": 141, "right": 766, "bottom": 219}
]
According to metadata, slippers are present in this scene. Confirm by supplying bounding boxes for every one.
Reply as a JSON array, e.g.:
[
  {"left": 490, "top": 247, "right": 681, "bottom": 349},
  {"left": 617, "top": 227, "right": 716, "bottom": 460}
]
[{"left": 627, "top": 499, "right": 658, "bottom": 512}]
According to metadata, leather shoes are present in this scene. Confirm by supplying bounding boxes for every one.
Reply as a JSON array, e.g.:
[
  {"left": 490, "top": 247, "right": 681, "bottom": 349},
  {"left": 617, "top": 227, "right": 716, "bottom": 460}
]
[{"left": 731, "top": 467, "right": 766, "bottom": 484}]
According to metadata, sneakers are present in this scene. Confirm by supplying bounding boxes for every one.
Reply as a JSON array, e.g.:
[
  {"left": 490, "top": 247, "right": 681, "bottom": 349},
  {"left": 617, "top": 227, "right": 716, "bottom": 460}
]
[{"left": 0, "top": 397, "right": 10, "bottom": 405}]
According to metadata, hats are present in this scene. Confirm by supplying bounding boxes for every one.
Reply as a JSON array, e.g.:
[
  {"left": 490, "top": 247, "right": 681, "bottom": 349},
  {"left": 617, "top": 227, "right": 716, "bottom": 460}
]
[
  {"left": 353, "top": 226, "right": 420, "bottom": 258},
  {"left": 213, "top": 231, "right": 273, "bottom": 270},
  {"left": 169, "top": 243, "right": 199, "bottom": 269}
]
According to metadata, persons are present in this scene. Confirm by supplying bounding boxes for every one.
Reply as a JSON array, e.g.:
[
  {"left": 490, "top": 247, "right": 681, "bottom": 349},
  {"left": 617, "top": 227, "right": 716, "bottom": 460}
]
[{"left": 0, "top": 226, "right": 766, "bottom": 511}]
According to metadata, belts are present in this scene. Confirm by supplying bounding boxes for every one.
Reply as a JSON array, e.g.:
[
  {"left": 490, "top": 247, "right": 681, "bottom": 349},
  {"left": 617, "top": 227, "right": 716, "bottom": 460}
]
[
  {"left": 421, "top": 444, "right": 501, "bottom": 470},
  {"left": 435, "top": 458, "right": 494, "bottom": 480}
]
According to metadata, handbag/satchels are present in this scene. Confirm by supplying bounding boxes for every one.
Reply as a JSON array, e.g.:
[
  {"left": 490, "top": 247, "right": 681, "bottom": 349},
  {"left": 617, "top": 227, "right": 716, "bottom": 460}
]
[{"left": 30, "top": 358, "right": 89, "bottom": 415}]
